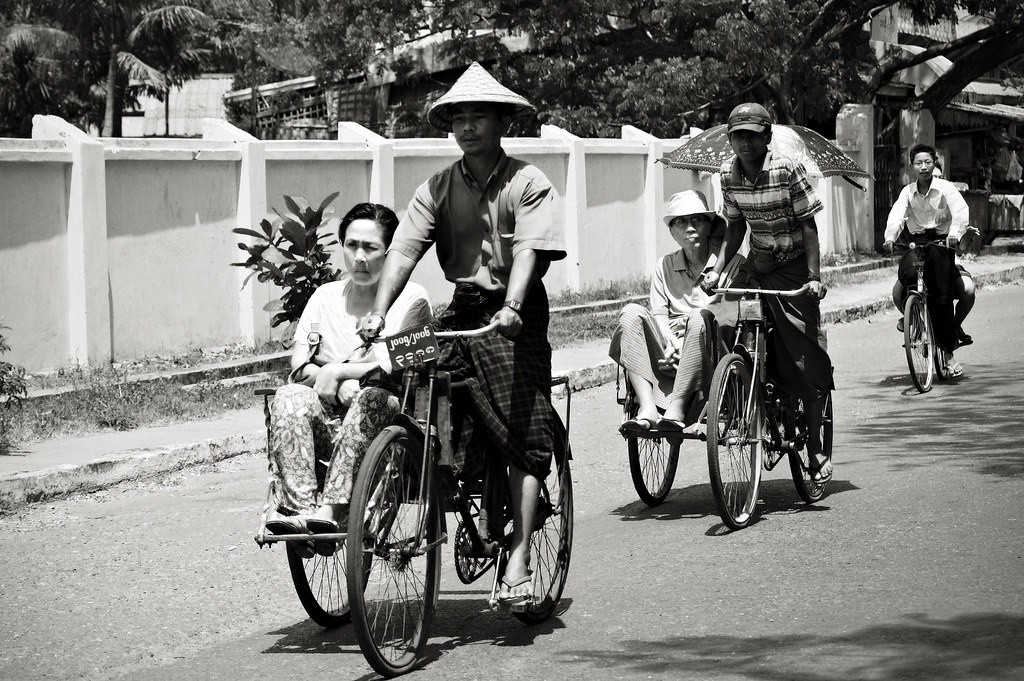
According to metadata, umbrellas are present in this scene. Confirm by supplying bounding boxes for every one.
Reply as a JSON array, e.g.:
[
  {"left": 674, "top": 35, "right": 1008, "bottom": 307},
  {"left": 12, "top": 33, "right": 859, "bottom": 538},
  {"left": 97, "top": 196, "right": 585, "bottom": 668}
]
[{"left": 653, "top": 125, "right": 877, "bottom": 193}]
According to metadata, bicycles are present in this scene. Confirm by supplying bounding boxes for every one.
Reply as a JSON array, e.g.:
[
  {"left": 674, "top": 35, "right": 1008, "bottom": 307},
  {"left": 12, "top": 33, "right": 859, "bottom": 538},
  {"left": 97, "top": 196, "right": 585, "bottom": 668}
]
[{"left": 880, "top": 234, "right": 959, "bottom": 393}]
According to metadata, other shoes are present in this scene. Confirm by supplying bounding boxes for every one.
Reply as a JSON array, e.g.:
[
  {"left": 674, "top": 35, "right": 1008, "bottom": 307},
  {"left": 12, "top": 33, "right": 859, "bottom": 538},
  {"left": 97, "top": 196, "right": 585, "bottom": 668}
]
[
  {"left": 656, "top": 416, "right": 685, "bottom": 446},
  {"left": 622, "top": 416, "right": 653, "bottom": 435},
  {"left": 954, "top": 335, "right": 973, "bottom": 350},
  {"left": 897, "top": 313, "right": 914, "bottom": 332},
  {"left": 306, "top": 510, "right": 340, "bottom": 556},
  {"left": 265, "top": 518, "right": 315, "bottom": 559}
]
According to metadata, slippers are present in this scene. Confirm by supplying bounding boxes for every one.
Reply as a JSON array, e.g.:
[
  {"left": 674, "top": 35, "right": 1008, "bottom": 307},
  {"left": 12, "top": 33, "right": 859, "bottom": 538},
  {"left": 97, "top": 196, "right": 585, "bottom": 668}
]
[
  {"left": 497, "top": 572, "right": 535, "bottom": 605},
  {"left": 947, "top": 363, "right": 963, "bottom": 377},
  {"left": 809, "top": 454, "right": 834, "bottom": 483}
]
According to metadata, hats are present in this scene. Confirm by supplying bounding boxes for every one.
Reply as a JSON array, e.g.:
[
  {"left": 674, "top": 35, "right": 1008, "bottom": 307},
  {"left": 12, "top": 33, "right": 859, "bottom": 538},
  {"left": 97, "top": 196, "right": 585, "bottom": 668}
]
[
  {"left": 663, "top": 190, "right": 716, "bottom": 224},
  {"left": 727, "top": 102, "right": 772, "bottom": 134},
  {"left": 427, "top": 60, "right": 536, "bottom": 132}
]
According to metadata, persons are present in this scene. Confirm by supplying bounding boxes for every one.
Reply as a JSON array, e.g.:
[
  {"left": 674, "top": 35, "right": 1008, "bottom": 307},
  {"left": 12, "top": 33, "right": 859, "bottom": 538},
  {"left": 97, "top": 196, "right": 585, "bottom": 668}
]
[
  {"left": 356, "top": 61, "right": 568, "bottom": 608},
  {"left": 881, "top": 146, "right": 980, "bottom": 378},
  {"left": 265, "top": 203, "right": 434, "bottom": 559},
  {"left": 700, "top": 104, "right": 835, "bottom": 484},
  {"left": 608, "top": 190, "right": 746, "bottom": 447}
]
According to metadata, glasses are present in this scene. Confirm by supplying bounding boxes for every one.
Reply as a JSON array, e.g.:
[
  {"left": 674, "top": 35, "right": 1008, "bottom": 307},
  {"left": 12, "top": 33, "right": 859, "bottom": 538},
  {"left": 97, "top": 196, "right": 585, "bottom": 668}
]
[
  {"left": 913, "top": 161, "right": 935, "bottom": 166},
  {"left": 673, "top": 216, "right": 705, "bottom": 228}
]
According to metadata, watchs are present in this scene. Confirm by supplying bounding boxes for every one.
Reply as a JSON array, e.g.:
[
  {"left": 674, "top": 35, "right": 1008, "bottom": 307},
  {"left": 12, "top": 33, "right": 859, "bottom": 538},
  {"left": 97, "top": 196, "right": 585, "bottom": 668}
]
[{"left": 502, "top": 299, "right": 522, "bottom": 312}]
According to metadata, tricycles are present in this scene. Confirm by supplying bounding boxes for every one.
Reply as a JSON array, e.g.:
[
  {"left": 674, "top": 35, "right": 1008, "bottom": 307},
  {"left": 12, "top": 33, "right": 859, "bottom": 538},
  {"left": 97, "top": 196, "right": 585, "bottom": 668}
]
[
  {"left": 615, "top": 271, "right": 834, "bottom": 530},
  {"left": 253, "top": 318, "right": 575, "bottom": 679}
]
